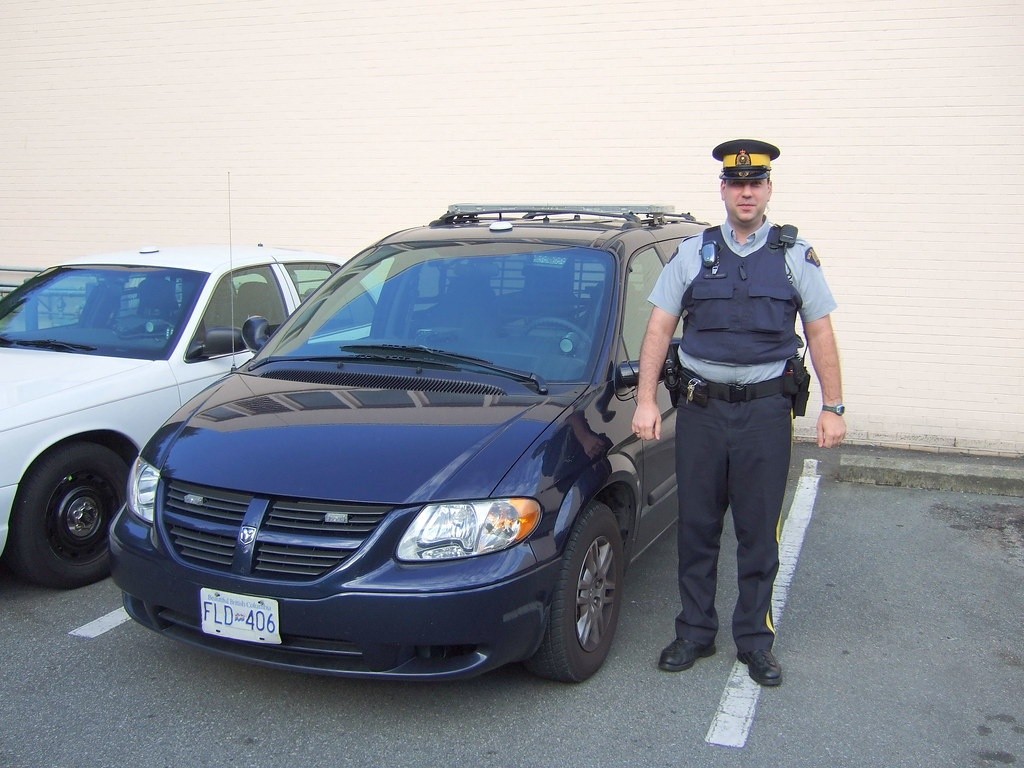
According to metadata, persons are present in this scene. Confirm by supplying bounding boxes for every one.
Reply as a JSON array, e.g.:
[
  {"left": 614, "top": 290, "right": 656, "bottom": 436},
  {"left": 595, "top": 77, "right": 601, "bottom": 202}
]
[
  {"left": 632, "top": 139, "right": 846, "bottom": 686},
  {"left": 532, "top": 409, "right": 614, "bottom": 503}
]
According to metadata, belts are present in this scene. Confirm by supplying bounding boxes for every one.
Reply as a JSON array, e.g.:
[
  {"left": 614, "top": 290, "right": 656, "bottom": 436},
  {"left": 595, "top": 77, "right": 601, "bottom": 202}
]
[{"left": 709, "top": 376, "right": 783, "bottom": 402}]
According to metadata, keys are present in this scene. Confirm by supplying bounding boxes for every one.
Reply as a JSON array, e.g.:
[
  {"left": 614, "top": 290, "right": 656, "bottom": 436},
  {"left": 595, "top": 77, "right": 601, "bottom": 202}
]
[{"left": 686, "top": 385, "right": 695, "bottom": 404}]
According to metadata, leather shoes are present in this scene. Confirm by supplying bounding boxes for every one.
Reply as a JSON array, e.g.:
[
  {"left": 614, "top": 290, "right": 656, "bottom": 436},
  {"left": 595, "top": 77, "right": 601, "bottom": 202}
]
[
  {"left": 658, "top": 637, "right": 716, "bottom": 671},
  {"left": 737, "top": 649, "right": 782, "bottom": 685}
]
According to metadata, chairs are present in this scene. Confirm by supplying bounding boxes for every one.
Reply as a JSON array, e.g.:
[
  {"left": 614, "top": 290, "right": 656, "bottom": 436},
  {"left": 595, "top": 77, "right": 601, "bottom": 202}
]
[
  {"left": 227, "top": 283, "right": 276, "bottom": 327},
  {"left": 424, "top": 275, "right": 497, "bottom": 356},
  {"left": 137, "top": 277, "right": 179, "bottom": 324}
]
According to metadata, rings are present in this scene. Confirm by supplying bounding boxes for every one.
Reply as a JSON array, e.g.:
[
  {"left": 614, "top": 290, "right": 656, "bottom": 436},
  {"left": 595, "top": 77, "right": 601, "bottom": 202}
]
[{"left": 636, "top": 431, "right": 640, "bottom": 435}]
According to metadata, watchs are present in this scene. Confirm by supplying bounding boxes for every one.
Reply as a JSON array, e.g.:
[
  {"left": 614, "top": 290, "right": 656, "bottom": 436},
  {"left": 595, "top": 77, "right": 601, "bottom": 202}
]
[{"left": 822, "top": 404, "right": 845, "bottom": 416}]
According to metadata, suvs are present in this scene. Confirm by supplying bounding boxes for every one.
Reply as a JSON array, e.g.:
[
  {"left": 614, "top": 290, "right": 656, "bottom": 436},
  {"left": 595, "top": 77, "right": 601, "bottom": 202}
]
[{"left": 105, "top": 203, "right": 713, "bottom": 689}]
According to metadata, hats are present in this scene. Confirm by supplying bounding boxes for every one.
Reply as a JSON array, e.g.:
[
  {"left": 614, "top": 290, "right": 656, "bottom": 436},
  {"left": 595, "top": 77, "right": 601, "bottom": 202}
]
[{"left": 713, "top": 139, "right": 780, "bottom": 179}]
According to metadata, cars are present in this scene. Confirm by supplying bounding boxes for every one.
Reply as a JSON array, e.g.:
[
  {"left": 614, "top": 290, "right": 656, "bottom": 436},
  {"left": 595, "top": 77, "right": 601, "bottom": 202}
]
[{"left": 0, "top": 246, "right": 382, "bottom": 593}]
